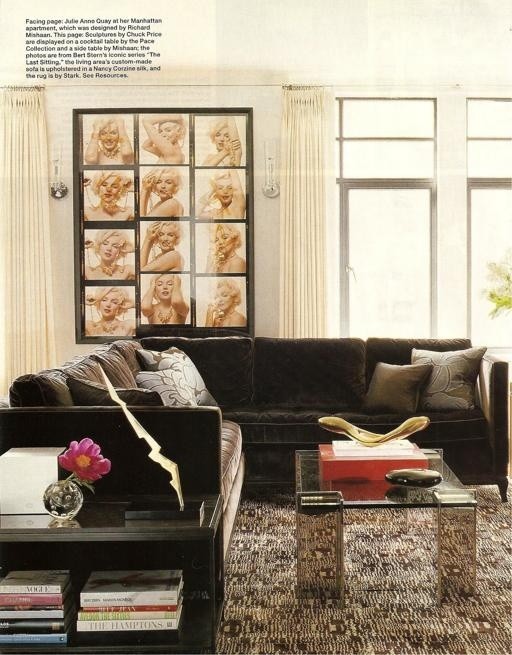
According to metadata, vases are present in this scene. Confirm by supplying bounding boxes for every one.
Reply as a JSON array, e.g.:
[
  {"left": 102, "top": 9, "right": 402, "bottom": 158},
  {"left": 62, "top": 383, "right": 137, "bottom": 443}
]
[
  {"left": 43, "top": 480, "right": 84, "bottom": 520},
  {"left": 58, "top": 438, "right": 111, "bottom": 494}
]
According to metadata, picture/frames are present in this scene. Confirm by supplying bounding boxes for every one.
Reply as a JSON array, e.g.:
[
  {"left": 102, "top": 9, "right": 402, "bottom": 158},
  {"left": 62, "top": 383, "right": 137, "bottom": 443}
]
[{"left": 72, "top": 108, "right": 254, "bottom": 342}]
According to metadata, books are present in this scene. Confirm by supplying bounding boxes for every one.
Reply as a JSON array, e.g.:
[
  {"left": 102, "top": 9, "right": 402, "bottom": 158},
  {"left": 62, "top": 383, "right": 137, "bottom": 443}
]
[{"left": 0, "top": 569, "right": 185, "bottom": 644}]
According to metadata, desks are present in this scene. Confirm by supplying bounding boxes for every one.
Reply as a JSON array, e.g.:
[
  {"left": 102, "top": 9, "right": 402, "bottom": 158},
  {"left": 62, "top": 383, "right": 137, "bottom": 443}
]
[{"left": 0, "top": 494, "right": 224, "bottom": 654}]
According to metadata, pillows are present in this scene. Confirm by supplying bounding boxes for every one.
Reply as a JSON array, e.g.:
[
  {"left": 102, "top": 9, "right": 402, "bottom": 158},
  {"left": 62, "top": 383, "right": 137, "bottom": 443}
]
[
  {"left": 66, "top": 344, "right": 221, "bottom": 408},
  {"left": 360, "top": 346, "right": 487, "bottom": 418}
]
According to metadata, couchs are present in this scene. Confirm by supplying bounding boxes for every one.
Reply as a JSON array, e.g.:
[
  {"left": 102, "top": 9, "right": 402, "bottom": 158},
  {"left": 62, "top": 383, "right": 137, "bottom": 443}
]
[{"left": 1, "top": 335, "right": 508, "bottom": 573}]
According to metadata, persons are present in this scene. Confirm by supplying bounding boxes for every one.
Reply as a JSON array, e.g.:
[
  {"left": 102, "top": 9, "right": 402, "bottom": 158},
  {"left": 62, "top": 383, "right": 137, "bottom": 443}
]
[
  {"left": 204, "top": 223, "right": 246, "bottom": 274},
  {"left": 197, "top": 169, "right": 246, "bottom": 220},
  {"left": 139, "top": 221, "right": 187, "bottom": 271},
  {"left": 204, "top": 278, "right": 247, "bottom": 327},
  {"left": 142, "top": 115, "right": 185, "bottom": 163},
  {"left": 140, "top": 167, "right": 184, "bottom": 217},
  {"left": 85, "top": 229, "right": 135, "bottom": 281},
  {"left": 201, "top": 113, "right": 242, "bottom": 167},
  {"left": 85, "top": 117, "right": 134, "bottom": 165},
  {"left": 84, "top": 286, "right": 136, "bottom": 336},
  {"left": 84, "top": 171, "right": 134, "bottom": 221},
  {"left": 142, "top": 274, "right": 190, "bottom": 325}
]
